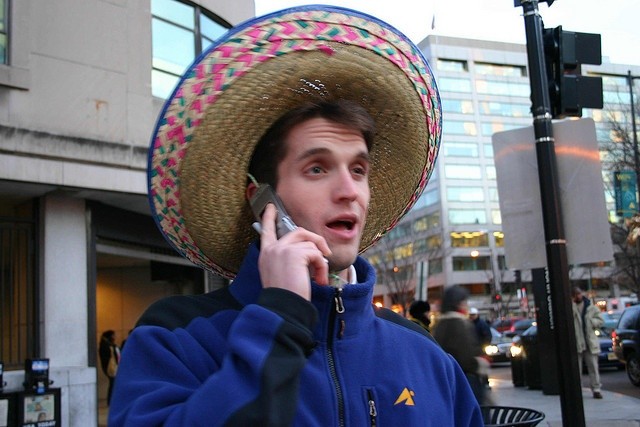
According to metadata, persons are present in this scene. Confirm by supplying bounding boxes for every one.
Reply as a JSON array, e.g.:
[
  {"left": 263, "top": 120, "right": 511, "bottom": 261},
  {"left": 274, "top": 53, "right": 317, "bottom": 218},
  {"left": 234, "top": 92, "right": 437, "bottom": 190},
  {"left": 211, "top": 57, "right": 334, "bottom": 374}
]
[
  {"left": 120, "top": 330, "right": 133, "bottom": 350},
  {"left": 468, "top": 307, "right": 492, "bottom": 390},
  {"left": 108, "top": 96, "right": 485, "bottom": 427},
  {"left": 434, "top": 284, "right": 484, "bottom": 406},
  {"left": 410, "top": 300, "right": 431, "bottom": 334},
  {"left": 571, "top": 287, "right": 604, "bottom": 399},
  {"left": 98, "top": 329, "right": 121, "bottom": 407}
]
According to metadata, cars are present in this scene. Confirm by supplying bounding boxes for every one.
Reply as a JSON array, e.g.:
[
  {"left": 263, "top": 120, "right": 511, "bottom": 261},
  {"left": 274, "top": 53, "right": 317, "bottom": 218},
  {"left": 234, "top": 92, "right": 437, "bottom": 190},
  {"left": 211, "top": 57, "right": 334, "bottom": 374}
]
[
  {"left": 482, "top": 327, "right": 512, "bottom": 363},
  {"left": 512, "top": 326, "right": 627, "bottom": 375}
]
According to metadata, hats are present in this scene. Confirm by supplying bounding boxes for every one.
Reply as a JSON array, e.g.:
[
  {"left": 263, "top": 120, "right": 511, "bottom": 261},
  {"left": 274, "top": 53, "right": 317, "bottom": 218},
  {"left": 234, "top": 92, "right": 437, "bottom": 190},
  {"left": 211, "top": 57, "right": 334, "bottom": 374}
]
[
  {"left": 148, "top": 4, "right": 442, "bottom": 281},
  {"left": 410, "top": 301, "right": 429, "bottom": 318}
]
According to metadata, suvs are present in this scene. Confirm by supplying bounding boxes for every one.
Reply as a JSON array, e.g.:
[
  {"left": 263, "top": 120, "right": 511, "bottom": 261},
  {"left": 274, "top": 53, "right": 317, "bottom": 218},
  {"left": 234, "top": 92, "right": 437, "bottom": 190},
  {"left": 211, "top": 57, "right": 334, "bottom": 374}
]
[{"left": 612, "top": 304, "right": 640, "bottom": 388}]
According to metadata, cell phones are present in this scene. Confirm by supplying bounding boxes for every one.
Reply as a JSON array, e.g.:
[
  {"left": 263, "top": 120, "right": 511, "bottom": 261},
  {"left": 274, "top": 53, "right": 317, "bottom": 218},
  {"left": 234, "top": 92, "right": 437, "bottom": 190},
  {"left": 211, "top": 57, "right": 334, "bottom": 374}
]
[{"left": 246, "top": 173, "right": 328, "bottom": 279}]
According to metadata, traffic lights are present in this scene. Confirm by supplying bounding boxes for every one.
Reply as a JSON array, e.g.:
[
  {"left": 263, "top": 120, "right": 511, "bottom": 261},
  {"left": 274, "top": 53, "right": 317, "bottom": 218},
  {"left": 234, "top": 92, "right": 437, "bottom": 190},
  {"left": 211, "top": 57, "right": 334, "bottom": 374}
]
[
  {"left": 494, "top": 293, "right": 502, "bottom": 302},
  {"left": 544, "top": 25, "right": 604, "bottom": 119}
]
